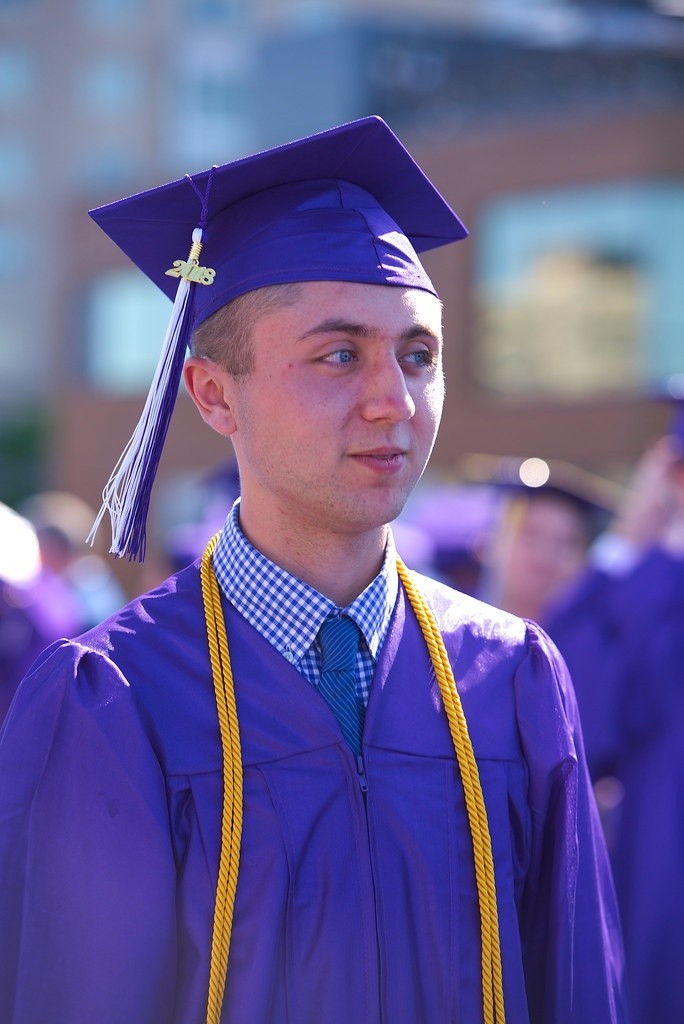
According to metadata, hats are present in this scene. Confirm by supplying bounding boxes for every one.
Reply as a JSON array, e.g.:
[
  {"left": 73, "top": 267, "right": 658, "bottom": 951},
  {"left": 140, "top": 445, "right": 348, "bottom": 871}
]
[
  {"left": 455, "top": 451, "right": 628, "bottom": 527},
  {"left": 87, "top": 116, "right": 467, "bottom": 551}
]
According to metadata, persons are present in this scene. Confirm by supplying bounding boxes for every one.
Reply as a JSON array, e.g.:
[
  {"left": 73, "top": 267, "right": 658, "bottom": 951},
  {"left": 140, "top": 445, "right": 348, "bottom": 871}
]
[
  {"left": 0, "top": 115, "right": 631, "bottom": 1024},
  {"left": 0, "top": 425, "right": 684, "bottom": 1024}
]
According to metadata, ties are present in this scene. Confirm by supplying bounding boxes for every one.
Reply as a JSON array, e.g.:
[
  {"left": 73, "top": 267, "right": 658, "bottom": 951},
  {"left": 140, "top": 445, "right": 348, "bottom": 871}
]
[{"left": 315, "top": 617, "right": 362, "bottom": 757}]
